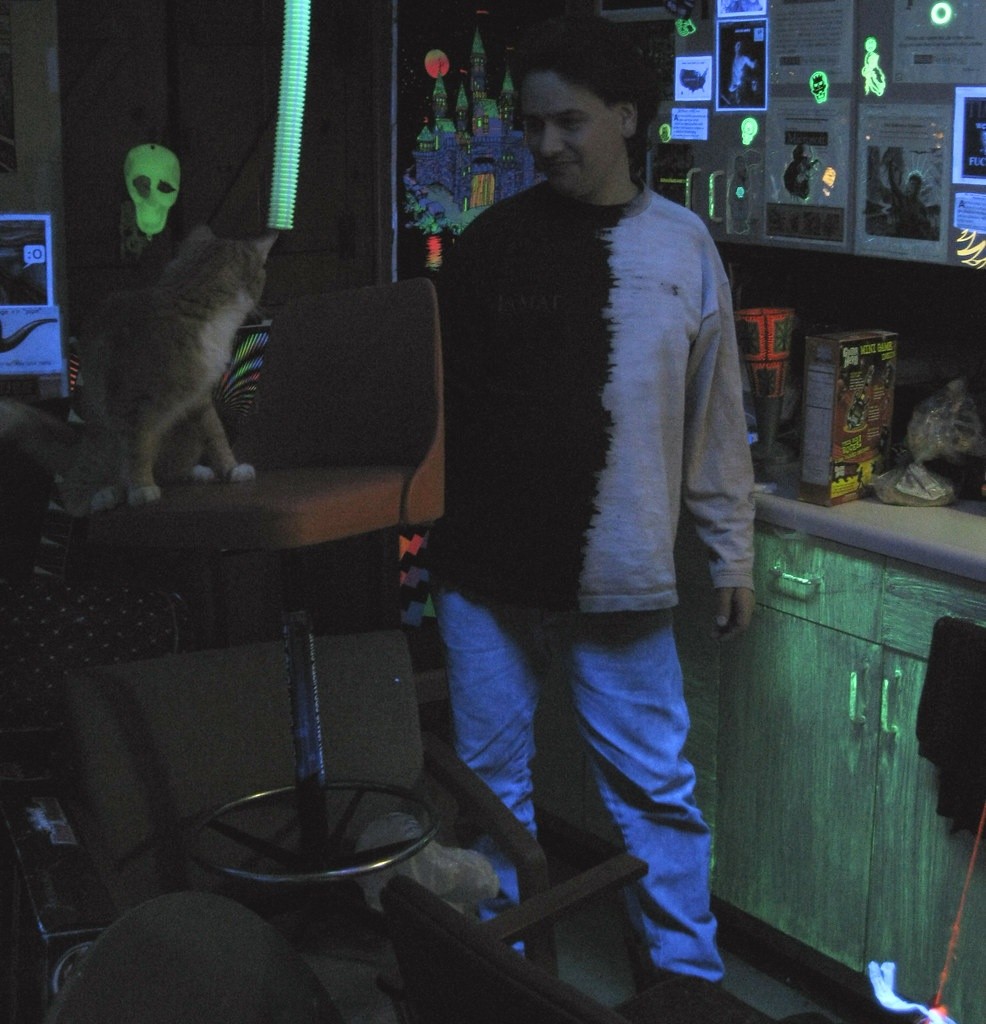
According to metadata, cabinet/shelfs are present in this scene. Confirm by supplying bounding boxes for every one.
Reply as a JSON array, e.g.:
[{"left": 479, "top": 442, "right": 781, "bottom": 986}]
[
  {"left": 565, "top": 0, "right": 986, "bottom": 267},
  {"left": 57, "top": 1, "right": 391, "bottom": 355},
  {"left": 527, "top": 521, "right": 985, "bottom": 1024}
]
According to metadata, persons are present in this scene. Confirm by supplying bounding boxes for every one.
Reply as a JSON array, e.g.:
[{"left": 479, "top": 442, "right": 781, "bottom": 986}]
[
  {"left": 728, "top": 39, "right": 758, "bottom": 106},
  {"left": 435, "top": 12, "right": 754, "bottom": 995}
]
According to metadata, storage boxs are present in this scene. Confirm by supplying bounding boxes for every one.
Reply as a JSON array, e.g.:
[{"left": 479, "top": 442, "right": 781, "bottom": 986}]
[{"left": 796, "top": 330, "right": 900, "bottom": 505}]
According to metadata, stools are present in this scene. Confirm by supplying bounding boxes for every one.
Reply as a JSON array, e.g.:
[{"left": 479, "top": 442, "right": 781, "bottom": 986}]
[{"left": 88, "top": 277, "right": 446, "bottom": 952}]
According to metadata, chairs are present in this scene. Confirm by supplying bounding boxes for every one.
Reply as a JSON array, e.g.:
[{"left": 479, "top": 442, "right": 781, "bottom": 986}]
[
  {"left": 382, "top": 852, "right": 836, "bottom": 1024},
  {"left": 1, "top": 632, "right": 556, "bottom": 1024}
]
